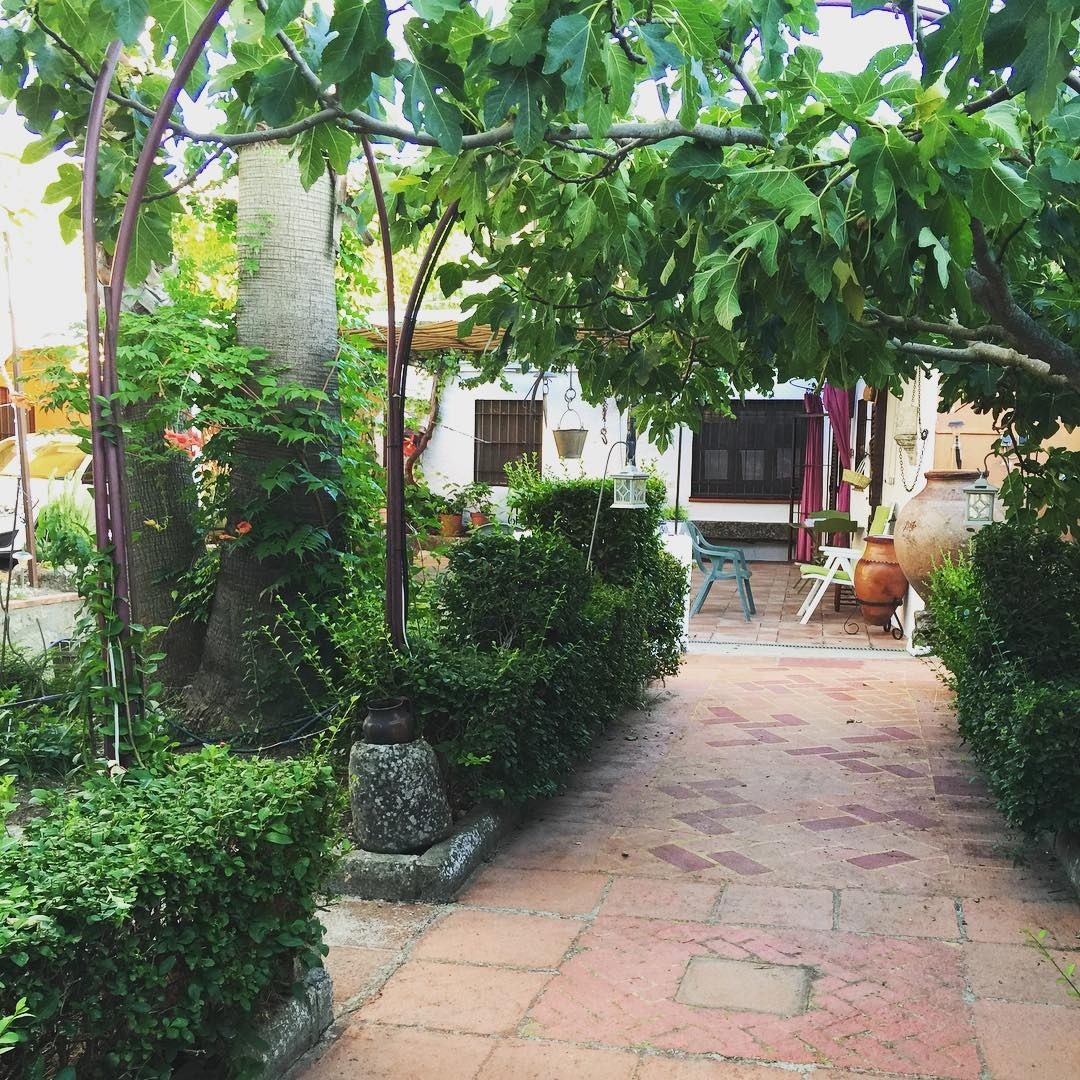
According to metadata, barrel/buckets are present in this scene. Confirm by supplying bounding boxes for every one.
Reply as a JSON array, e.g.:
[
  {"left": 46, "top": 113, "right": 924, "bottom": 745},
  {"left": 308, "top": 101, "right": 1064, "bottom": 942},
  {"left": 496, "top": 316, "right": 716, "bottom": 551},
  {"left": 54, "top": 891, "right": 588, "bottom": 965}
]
[{"left": 552, "top": 409, "right": 589, "bottom": 458}]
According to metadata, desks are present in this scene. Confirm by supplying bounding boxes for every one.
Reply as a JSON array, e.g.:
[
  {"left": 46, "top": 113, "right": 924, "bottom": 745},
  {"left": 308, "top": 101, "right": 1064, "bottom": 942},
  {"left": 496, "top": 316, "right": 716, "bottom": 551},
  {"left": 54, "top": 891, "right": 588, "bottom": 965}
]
[{"left": 789, "top": 523, "right": 865, "bottom": 593}]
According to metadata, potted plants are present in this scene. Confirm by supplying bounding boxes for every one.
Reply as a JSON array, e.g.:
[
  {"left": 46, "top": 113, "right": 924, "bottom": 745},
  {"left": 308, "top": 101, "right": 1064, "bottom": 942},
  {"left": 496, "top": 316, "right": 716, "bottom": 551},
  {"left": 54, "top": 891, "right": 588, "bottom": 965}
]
[{"left": 438, "top": 481, "right": 499, "bottom": 539}]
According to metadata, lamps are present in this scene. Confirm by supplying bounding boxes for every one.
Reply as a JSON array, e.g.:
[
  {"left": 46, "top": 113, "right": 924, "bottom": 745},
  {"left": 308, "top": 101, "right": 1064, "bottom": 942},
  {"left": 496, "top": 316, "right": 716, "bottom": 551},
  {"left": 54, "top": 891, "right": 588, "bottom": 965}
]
[
  {"left": 609, "top": 458, "right": 650, "bottom": 509},
  {"left": 963, "top": 469, "right": 999, "bottom": 523},
  {"left": 855, "top": 535, "right": 908, "bottom": 627}
]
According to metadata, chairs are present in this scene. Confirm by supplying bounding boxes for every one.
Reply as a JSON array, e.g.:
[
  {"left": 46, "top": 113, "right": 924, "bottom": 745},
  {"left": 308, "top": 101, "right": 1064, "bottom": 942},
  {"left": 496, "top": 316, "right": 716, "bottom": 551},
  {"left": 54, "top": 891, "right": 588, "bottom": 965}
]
[
  {"left": 686, "top": 520, "right": 756, "bottom": 622},
  {"left": 792, "top": 503, "right": 895, "bottom": 624}
]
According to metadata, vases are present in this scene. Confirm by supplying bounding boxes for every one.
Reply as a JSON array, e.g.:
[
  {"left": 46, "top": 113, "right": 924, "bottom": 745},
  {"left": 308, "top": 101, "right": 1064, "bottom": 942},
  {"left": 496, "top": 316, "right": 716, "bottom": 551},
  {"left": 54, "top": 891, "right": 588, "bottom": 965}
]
[
  {"left": 894, "top": 469, "right": 1008, "bottom": 607},
  {"left": 363, "top": 696, "right": 415, "bottom": 744}
]
